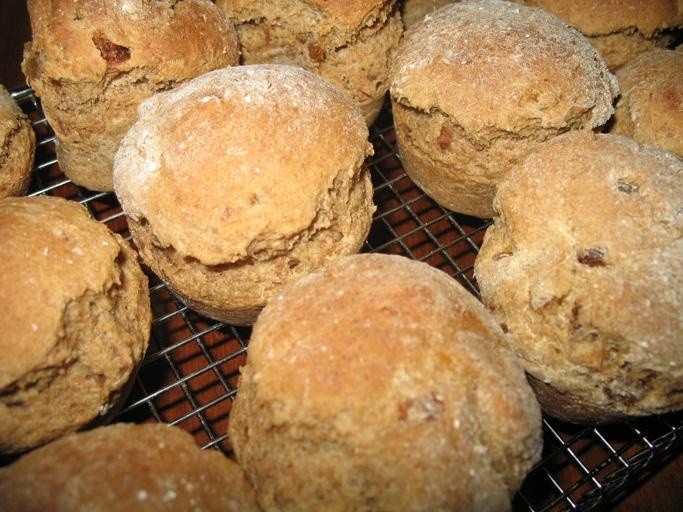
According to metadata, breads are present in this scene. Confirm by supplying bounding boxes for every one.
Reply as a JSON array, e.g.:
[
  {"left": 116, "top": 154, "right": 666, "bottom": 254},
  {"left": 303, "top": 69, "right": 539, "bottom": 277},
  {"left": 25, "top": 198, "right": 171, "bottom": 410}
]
[{"left": 1, "top": 0, "right": 683, "bottom": 512}]
